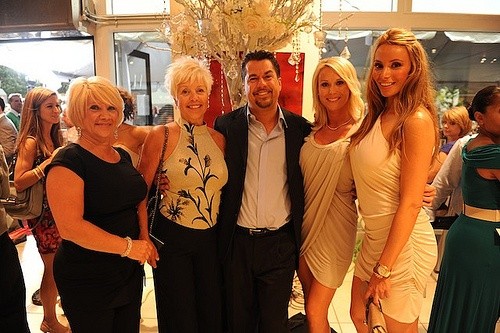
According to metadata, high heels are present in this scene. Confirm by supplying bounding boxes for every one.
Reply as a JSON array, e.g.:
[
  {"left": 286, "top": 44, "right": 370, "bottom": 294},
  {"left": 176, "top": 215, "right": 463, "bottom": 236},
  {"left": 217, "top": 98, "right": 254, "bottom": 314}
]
[{"left": 40, "top": 320, "right": 73, "bottom": 333}]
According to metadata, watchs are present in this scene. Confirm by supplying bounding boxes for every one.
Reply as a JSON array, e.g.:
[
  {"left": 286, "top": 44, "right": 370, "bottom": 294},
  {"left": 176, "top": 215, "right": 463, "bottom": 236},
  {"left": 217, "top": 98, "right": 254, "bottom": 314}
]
[{"left": 373, "top": 261, "right": 392, "bottom": 280}]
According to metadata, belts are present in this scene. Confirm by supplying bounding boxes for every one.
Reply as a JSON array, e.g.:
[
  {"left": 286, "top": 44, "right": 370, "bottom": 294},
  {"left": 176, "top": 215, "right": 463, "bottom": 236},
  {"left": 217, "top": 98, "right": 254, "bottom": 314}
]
[{"left": 236, "top": 219, "right": 293, "bottom": 238}]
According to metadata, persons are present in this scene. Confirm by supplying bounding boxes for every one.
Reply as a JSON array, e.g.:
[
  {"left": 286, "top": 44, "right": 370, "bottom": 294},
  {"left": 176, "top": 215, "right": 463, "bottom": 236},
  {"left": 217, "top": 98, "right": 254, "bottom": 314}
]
[
  {"left": 14, "top": 88, "right": 73, "bottom": 333},
  {"left": 427, "top": 85, "right": 500, "bottom": 333},
  {"left": 46, "top": 76, "right": 160, "bottom": 333},
  {"left": 296, "top": 56, "right": 437, "bottom": 333},
  {"left": 153, "top": 50, "right": 357, "bottom": 333},
  {"left": 137, "top": 56, "right": 228, "bottom": 333},
  {"left": 0, "top": 86, "right": 174, "bottom": 161},
  {"left": 0, "top": 146, "right": 32, "bottom": 333},
  {"left": 428, "top": 107, "right": 479, "bottom": 283},
  {"left": 349, "top": 27, "right": 440, "bottom": 333}
]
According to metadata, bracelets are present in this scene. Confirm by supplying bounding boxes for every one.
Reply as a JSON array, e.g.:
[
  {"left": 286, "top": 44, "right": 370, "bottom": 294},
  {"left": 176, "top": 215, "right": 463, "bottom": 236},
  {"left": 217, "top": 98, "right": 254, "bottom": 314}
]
[
  {"left": 121, "top": 236, "right": 133, "bottom": 261},
  {"left": 32, "top": 165, "right": 45, "bottom": 180}
]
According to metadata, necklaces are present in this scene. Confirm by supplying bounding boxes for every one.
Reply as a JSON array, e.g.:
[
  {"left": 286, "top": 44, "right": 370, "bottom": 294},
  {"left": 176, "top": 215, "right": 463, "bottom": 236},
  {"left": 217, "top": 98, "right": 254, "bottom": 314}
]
[{"left": 325, "top": 116, "right": 352, "bottom": 130}]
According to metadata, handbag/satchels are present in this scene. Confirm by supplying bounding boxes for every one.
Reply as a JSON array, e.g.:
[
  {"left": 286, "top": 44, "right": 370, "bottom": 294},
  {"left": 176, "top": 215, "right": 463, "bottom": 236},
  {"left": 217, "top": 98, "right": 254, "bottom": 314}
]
[
  {"left": 149, "top": 234, "right": 164, "bottom": 251},
  {"left": 0, "top": 136, "right": 43, "bottom": 219},
  {"left": 360, "top": 280, "right": 387, "bottom": 333}
]
